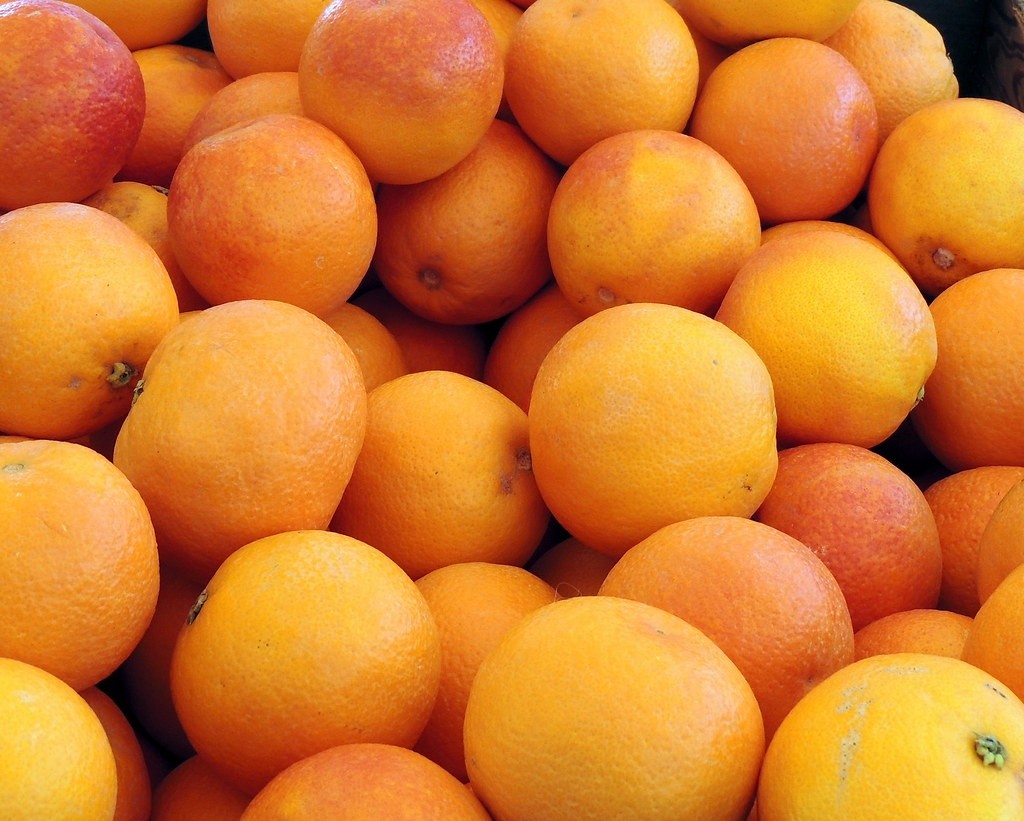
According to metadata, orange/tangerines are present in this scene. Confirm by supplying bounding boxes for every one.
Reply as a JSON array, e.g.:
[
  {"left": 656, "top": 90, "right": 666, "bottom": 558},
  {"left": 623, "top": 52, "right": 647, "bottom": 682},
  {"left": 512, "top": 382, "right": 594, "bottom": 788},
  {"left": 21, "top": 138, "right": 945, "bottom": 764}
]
[{"left": 0, "top": 0, "right": 1024, "bottom": 821}]
